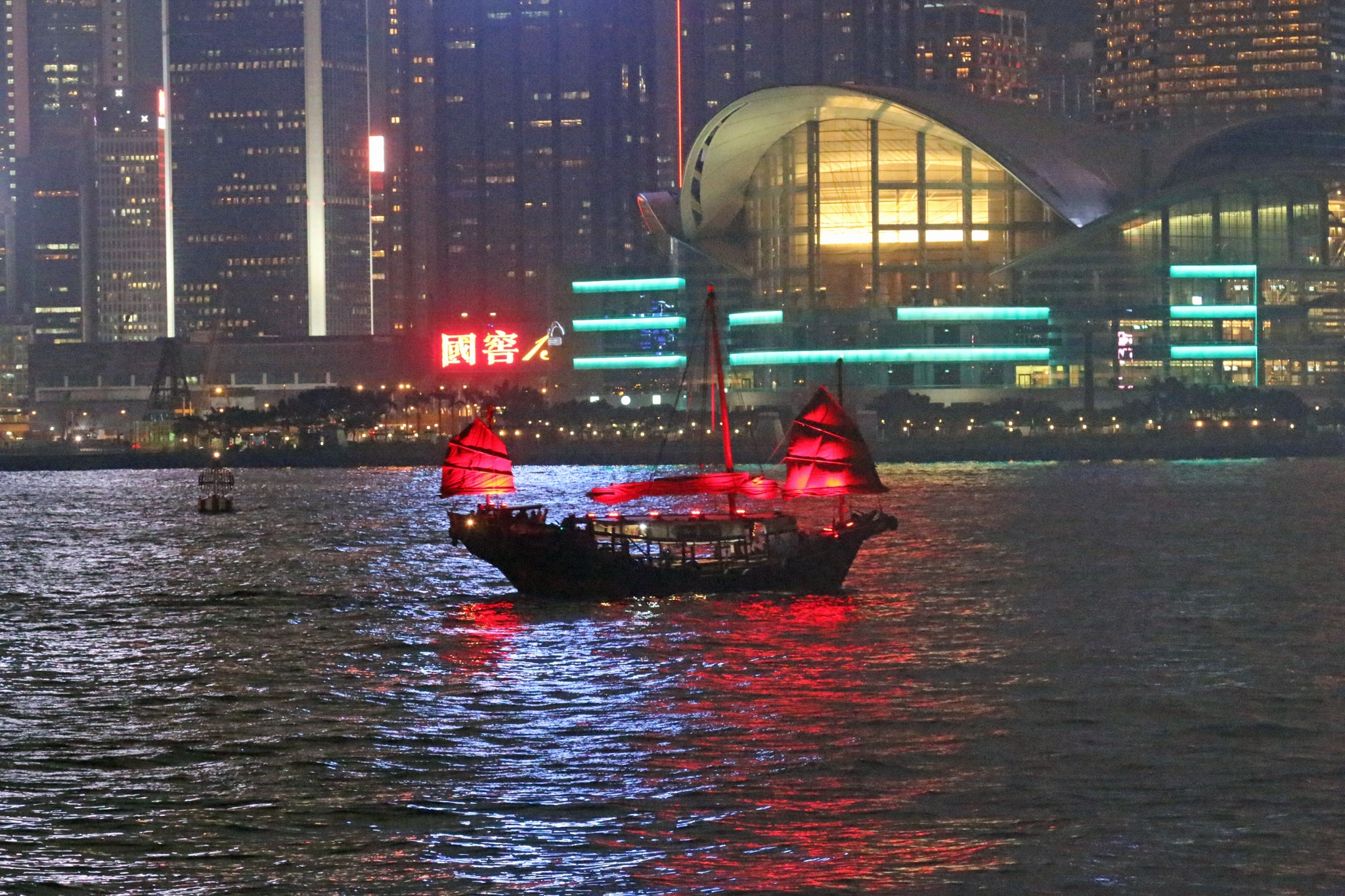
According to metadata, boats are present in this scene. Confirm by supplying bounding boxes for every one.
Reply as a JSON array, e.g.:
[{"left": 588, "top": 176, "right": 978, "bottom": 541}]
[{"left": 195, "top": 462, "right": 236, "bottom": 515}]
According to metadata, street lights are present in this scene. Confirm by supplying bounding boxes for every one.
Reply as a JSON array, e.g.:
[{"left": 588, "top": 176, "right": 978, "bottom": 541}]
[
  {"left": 1079, "top": 416, "right": 1087, "bottom": 437},
  {"left": 463, "top": 384, "right": 477, "bottom": 427},
  {"left": 935, "top": 419, "right": 942, "bottom": 438},
  {"left": 372, "top": 385, "right": 396, "bottom": 440},
  {"left": 587, "top": 423, "right": 591, "bottom": 441},
  {"left": 1112, "top": 417, "right": 1119, "bottom": 437},
  {"left": 967, "top": 419, "right": 974, "bottom": 438},
  {"left": 1315, "top": 406, "right": 1319, "bottom": 424},
  {"left": 692, "top": 421, "right": 695, "bottom": 436},
  {"left": 613, "top": 423, "right": 616, "bottom": 438},
  {"left": 659, "top": 426, "right": 663, "bottom": 439},
  {"left": 719, "top": 422, "right": 722, "bottom": 434},
  {"left": 560, "top": 427, "right": 564, "bottom": 446},
  {"left": 1008, "top": 421, "right": 1013, "bottom": 439},
  {"left": 633, "top": 422, "right": 637, "bottom": 439},
  {"left": 546, "top": 422, "right": 550, "bottom": 439},
  {"left": 439, "top": 386, "right": 445, "bottom": 416},
  {"left": 903, "top": 420, "right": 911, "bottom": 437},
  {"left": 1254, "top": 408, "right": 1258, "bottom": 420},
  {"left": 747, "top": 421, "right": 751, "bottom": 435},
  {"left": 1148, "top": 419, "right": 1153, "bottom": 438},
  {"left": 1190, "top": 410, "right": 1193, "bottom": 425},
  {"left": 398, "top": 384, "right": 419, "bottom": 441},
  {"left": 528, "top": 421, "right": 533, "bottom": 436},
  {"left": 1046, "top": 417, "right": 1054, "bottom": 436}
]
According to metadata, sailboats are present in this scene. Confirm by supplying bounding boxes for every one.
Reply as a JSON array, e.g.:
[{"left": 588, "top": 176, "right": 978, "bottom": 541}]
[{"left": 438, "top": 287, "right": 898, "bottom": 603}]
